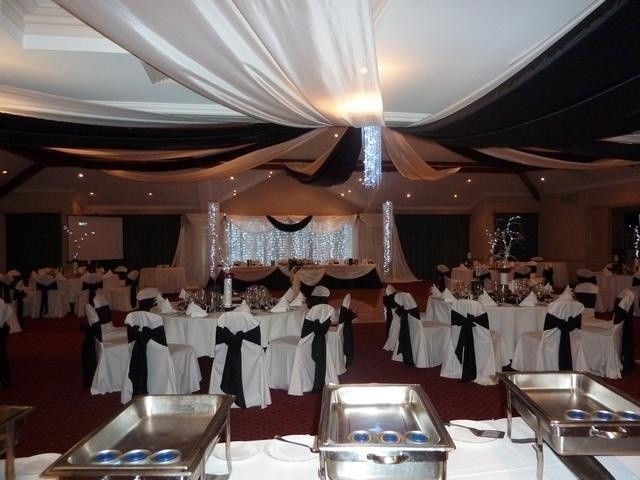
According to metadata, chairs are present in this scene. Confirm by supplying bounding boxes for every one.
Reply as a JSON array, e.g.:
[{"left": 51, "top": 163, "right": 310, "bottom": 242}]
[
  {"left": 74, "top": 274, "right": 104, "bottom": 317},
  {"left": 121, "top": 311, "right": 202, "bottom": 405},
  {"left": 603, "top": 263, "right": 629, "bottom": 274},
  {"left": 532, "top": 257, "right": 544, "bottom": 262},
  {"left": 391, "top": 292, "right": 452, "bottom": 368},
  {"left": 8, "top": 270, "right": 32, "bottom": 291},
  {"left": 632, "top": 270, "right": 640, "bottom": 317},
  {"left": 510, "top": 301, "right": 590, "bottom": 372},
  {"left": 526, "top": 261, "right": 537, "bottom": 278},
  {"left": 435, "top": 265, "right": 451, "bottom": 292},
  {"left": 208, "top": 311, "right": 272, "bottom": 409},
  {"left": 38, "top": 267, "right": 58, "bottom": 274},
  {"left": 542, "top": 263, "right": 554, "bottom": 288},
  {"left": 382, "top": 284, "right": 427, "bottom": 352},
  {"left": 512, "top": 266, "right": 531, "bottom": 287},
  {"left": 473, "top": 266, "right": 491, "bottom": 288},
  {"left": 452, "top": 268, "right": 530, "bottom": 293},
  {"left": 578, "top": 295, "right": 633, "bottom": 379},
  {"left": 79, "top": 303, "right": 129, "bottom": 395},
  {"left": 577, "top": 269, "right": 605, "bottom": 312},
  {"left": 94, "top": 294, "right": 127, "bottom": 341},
  {"left": 114, "top": 267, "right": 127, "bottom": 286},
  {"left": 0, "top": 273, "right": 33, "bottom": 316},
  {"left": 32, "top": 274, "right": 71, "bottom": 318},
  {"left": 329, "top": 293, "right": 358, "bottom": 376},
  {"left": 582, "top": 288, "right": 635, "bottom": 329},
  {"left": 136, "top": 288, "right": 162, "bottom": 312},
  {"left": 572, "top": 283, "right": 598, "bottom": 319},
  {"left": 109, "top": 271, "right": 139, "bottom": 311},
  {"left": 440, "top": 299, "right": 511, "bottom": 385},
  {"left": 308, "top": 286, "right": 330, "bottom": 310},
  {"left": 265, "top": 304, "right": 339, "bottom": 395}
]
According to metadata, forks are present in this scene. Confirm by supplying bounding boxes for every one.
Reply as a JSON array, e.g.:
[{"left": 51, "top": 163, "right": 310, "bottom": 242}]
[{"left": 440, "top": 420, "right": 505, "bottom": 439}]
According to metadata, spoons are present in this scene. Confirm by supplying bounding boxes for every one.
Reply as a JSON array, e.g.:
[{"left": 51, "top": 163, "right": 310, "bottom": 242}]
[{"left": 274, "top": 435, "right": 321, "bottom": 453}]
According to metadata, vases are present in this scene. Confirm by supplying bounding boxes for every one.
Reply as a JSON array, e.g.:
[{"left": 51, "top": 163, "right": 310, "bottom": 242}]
[
  {"left": 495, "top": 267, "right": 513, "bottom": 303},
  {"left": 498, "top": 262, "right": 510, "bottom": 303},
  {"left": 223, "top": 273, "right": 233, "bottom": 310}
]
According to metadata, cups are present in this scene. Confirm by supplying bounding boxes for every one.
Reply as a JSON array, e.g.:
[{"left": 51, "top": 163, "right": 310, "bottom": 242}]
[
  {"left": 453, "top": 280, "right": 552, "bottom": 305},
  {"left": 183, "top": 284, "right": 277, "bottom": 310}
]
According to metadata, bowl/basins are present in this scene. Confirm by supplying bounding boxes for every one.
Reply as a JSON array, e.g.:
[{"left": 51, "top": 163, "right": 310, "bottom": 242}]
[
  {"left": 313, "top": 383, "right": 456, "bottom": 479},
  {"left": 494, "top": 371, "right": 639, "bottom": 455},
  {"left": 38, "top": 394, "right": 230, "bottom": 480}
]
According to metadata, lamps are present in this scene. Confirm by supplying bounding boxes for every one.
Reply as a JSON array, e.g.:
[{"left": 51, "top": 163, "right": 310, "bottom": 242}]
[{"left": 361, "top": 125, "right": 383, "bottom": 191}]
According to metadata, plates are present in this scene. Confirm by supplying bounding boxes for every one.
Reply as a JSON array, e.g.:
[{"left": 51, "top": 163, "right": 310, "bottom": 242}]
[
  {"left": 16, "top": 453, "right": 62, "bottom": 474},
  {"left": 266, "top": 435, "right": 319, "bottom": 461},
  {"left": 443, "top": 419, "right": 499, "bottom": 443},
  {"left": 215, "top": 441, "right": 261, "bottom": 460}
]
